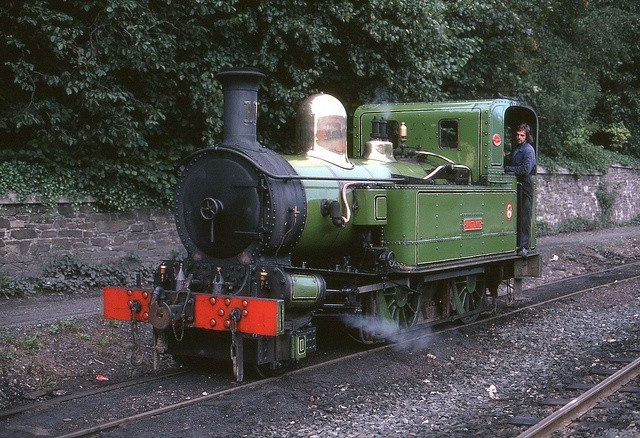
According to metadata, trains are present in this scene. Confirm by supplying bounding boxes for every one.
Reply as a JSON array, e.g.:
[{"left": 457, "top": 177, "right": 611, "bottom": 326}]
[{"left": 103, "top": 70, "right": 543, "bottom": 382}]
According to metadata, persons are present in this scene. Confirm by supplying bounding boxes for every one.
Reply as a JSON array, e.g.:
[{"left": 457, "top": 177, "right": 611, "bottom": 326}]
[{"left": 504, "top": 122, "right": 537, "bottom": 256}]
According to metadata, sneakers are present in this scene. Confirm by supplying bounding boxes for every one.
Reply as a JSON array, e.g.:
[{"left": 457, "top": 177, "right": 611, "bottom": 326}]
[{"left": 517, "top": 247, "right": 529, "bottom": 257}]
